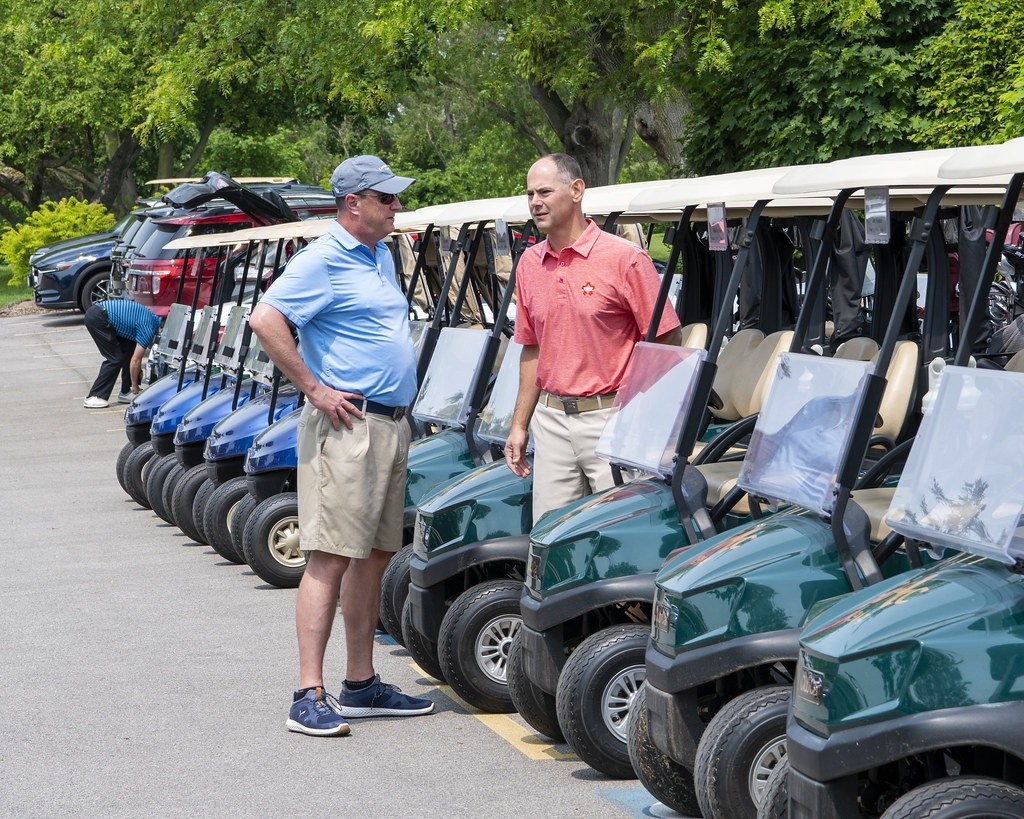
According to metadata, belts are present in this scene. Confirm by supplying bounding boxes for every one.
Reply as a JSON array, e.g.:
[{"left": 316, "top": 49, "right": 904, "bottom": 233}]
[
  {"left": 538, "top": 393, "right": 616, "bottom": 414},
  {"left": 347, "top": 399, "right": 408, "bottom": 421}
]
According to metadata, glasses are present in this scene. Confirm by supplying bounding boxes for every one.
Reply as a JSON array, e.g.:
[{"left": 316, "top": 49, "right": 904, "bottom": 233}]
[{"left": 356, "top": 193, "right": 398, "bottom": 204}]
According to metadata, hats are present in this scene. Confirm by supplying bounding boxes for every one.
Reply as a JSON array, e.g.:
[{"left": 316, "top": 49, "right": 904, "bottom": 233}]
[{"left": 331, "top": 155, "right": 416, "bottom": 198}]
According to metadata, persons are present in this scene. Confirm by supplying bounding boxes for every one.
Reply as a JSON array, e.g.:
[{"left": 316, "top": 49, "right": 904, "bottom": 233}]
[
  {"left": 250, "top": 156, "right": 435, "bottom": 736},
  {"left": 84, "top": 300, "right": 166, "bottom": 408},
  {"left": 503, "top": 153, "right": 684, "bottom": 589},
  {"left": 947, "top": 252, "right": 963, "bottom": 352}
]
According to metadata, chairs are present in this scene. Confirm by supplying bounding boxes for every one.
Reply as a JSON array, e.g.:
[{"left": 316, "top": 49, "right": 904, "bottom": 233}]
[{"left": 446, "top": 322, "right": 1024, "bottom": 560}]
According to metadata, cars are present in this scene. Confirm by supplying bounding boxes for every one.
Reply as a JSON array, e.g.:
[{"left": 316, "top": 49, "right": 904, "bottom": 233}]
[{"left": 27, "top": 195, "right": 160, "bottom": 316}]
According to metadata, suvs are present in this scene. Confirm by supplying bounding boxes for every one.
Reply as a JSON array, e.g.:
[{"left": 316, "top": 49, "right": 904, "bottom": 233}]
[{"left": 108, "top": 186, "right": 419, "bottom": 358}]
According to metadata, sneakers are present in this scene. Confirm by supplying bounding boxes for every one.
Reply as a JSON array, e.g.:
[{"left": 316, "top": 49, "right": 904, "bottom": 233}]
[
  {"left": 336, "top": 674, "right": 434, "bottom": 718},
  {"left": 286, "top": 688, "right": 351, "bottom": 736},
  {"left": 118, "top": 391, "right": 139, "bottom": 404},
  {"left": 83, "top": 396, "right": 108, "bottom": 408}
]
